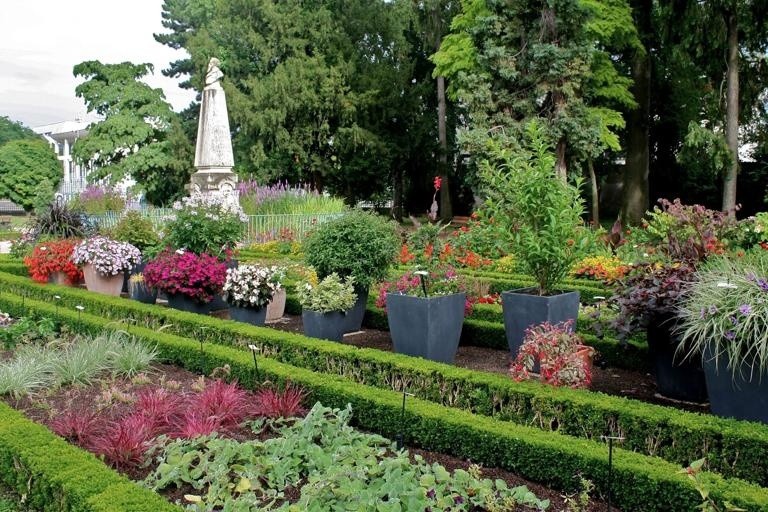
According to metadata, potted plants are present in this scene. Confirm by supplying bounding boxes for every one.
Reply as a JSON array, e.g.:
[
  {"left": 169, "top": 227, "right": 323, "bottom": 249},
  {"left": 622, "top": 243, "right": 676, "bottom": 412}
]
[
  {"left": 584, "top": 195, "right": 742, "bottom": 407},
  {"left": 383, "top": 220, "right": 468, "bottom": 366},
  {"left": 303, "top": 207, "right": 402, "bottom": 334},
  {"left": 266, "top": 271, "right": 295, "bottom": 321},
  {"left": 659, "top": 249, "right": 768, "bottom": 425},
  {"left": 296, "top": 264, "right": 358, "bottom": 344},
  {"left": 463, "top": 117, "right": 581, "bottom": 375}
]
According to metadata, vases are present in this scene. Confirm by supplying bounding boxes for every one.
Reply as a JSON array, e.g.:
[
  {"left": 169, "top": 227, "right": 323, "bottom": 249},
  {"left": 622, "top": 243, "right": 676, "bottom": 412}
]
[
  {"left": 167, "top": 291, "right": 211, "bottom": 317},
  {"left": 227, "top": 301, "right": 267, "bottom": 325},
  {"left": 81, "top": 263, "right": 125, "bottom": 298},
  {"left": 47, "top": 268, "right": 67, "bottom": 287}
]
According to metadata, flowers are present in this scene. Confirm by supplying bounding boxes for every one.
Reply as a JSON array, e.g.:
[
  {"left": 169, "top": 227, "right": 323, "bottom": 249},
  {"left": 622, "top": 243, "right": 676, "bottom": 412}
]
[
  {"left": 70, "top": 235, "right": 144, "bottom": 278},
  {"left": 142, "top": 245, "right": 238, "bottom": 303},
  {"left": 21, "top": 238, "right": 82, "bottom": 287},
  {"left": 221, "top": 259, "right": 282, "bottom": 311}
]
